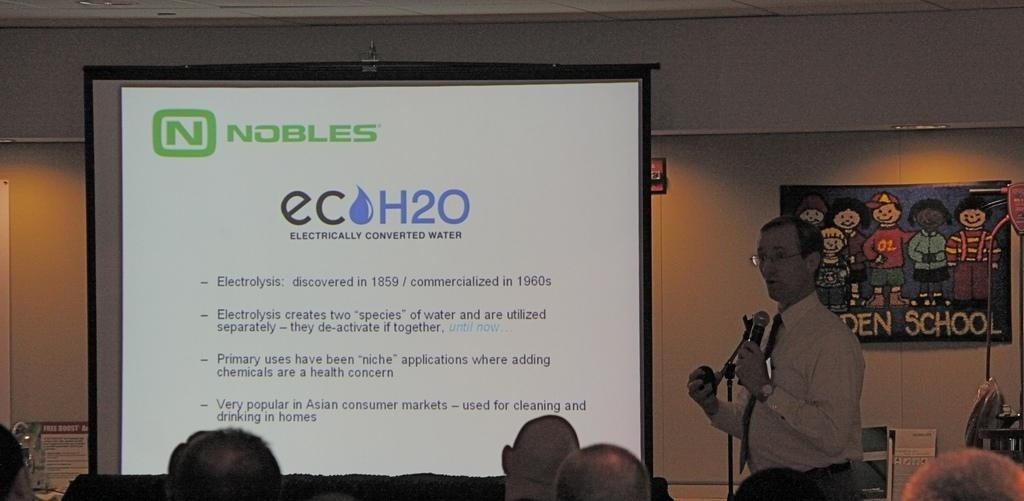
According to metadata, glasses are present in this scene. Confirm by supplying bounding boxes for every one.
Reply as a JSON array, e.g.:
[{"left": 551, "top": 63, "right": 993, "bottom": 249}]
[{"left": 750, "top": 252, "right": 799, "bottom": 265}]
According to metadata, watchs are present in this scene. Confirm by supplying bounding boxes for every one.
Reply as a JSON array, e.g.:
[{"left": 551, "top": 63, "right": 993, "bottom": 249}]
[{"left": 757, "top": 384, "right": 775, "bottom": 403}]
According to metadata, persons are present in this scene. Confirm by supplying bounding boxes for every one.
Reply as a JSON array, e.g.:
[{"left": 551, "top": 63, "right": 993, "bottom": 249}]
[
  {"left": 500, "top": 417, "right": 577, "bottom": 500},
  {"left": 1, "top": 421, "right": 36, "bottom": 501},
  {"left": 900, "top": 452, "right": 1024, "bottom": 501},
  {"left": 734, "top": 467, "right": 822, "bottom": 501},
  {"left": 684, "top": 216, "right": 867, "bottom": 501},
  {"left": 161, "top": 430, "right": 208, "bottom": 500},
  {"left": 554, "top": 441, "right": 651, "bottom": 501},
  {"left": 176, "top": 426, "right": 281, "bottom": 500}
]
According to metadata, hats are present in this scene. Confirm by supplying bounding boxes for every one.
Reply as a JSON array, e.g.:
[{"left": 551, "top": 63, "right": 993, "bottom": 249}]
[{"left": 864, "top": 192, "right": 900, "bottom": 208}]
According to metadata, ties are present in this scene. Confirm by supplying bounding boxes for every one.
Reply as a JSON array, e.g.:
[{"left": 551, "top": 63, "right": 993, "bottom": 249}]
[{"left": 739, "top": 315, "right": 781, "bottom": 474}]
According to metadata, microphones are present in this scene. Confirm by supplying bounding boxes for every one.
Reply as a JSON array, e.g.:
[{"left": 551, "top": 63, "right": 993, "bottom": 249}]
[{"left": 736, "top": 306, "right": 770, "bottom": 384}]
